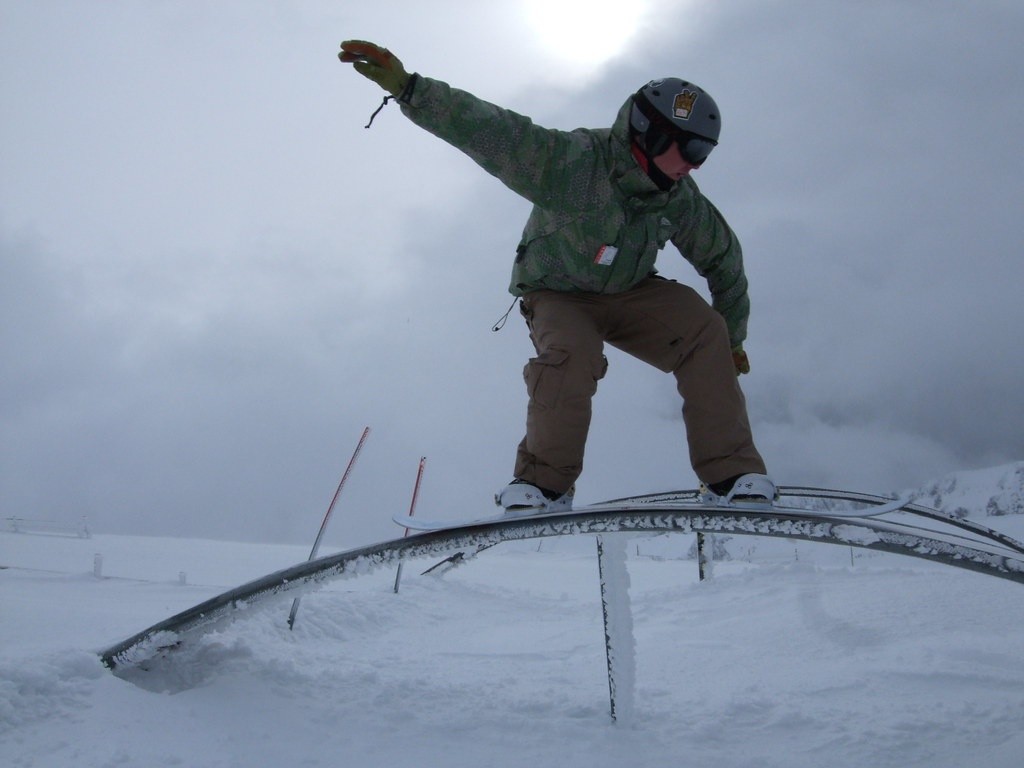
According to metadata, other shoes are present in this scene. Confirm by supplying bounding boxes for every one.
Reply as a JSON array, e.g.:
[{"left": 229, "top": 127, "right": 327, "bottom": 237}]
[
  {"left": 510, "top": 478, "right": 560, "bottom": 510},
  {"left": 708, "top": 473, "right": 762, "bottom": 499}
]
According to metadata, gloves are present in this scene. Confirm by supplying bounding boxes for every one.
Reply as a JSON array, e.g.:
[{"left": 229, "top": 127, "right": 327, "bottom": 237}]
[
  {"left": 733, "top": 344, "right": 749, "bottom": 376},
  {"left": 338, "top": 40, "right": 410, "bottom": 101}
]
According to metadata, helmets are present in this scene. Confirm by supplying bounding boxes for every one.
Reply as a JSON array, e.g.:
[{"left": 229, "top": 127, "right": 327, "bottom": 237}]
[{"left": 630, "top": 77, "right": 721, "bottom": 155}]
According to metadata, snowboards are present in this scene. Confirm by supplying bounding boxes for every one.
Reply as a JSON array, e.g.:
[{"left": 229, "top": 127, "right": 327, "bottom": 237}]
[{"left": 388, "top": 489, "right": 920, "bottom": 534}]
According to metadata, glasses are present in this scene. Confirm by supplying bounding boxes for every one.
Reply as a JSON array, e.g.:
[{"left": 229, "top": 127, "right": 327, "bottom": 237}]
[{"left": 678, "top": 134, "right": 716, "bottom": 169}]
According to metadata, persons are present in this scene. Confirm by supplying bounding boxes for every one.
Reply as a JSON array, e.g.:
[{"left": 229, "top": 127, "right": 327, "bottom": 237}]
[{"left": 338, "top": 39, "right": 770, "bottom": 509}]
